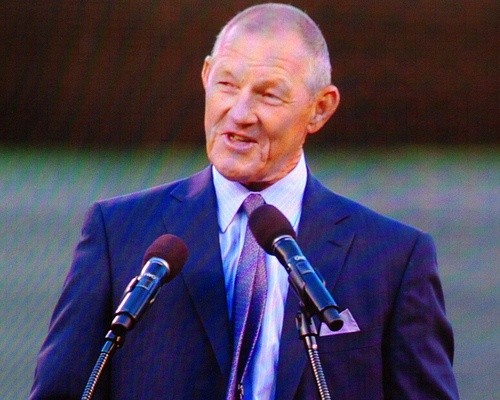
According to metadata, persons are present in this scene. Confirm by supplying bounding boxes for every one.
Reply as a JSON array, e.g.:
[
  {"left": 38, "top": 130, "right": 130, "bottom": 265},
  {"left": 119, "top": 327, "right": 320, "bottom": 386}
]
[{"left": 26, "top": 2, "right": 459, "bottom": 400}]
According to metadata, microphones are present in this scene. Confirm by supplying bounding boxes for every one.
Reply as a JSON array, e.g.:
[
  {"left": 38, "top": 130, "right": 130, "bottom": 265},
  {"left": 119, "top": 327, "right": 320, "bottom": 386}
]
[
  {"left": 101, "top": 233, "right": 189, "bottom": 356},
  {"left": 248, "top": 204, "right": 344, "bottom": 332}
]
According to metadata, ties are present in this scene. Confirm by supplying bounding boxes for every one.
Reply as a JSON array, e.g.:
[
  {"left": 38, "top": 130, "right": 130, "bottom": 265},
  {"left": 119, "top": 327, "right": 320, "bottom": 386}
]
[{"left": 226, "top": 194, "right": 267, "bottom": 399}]
